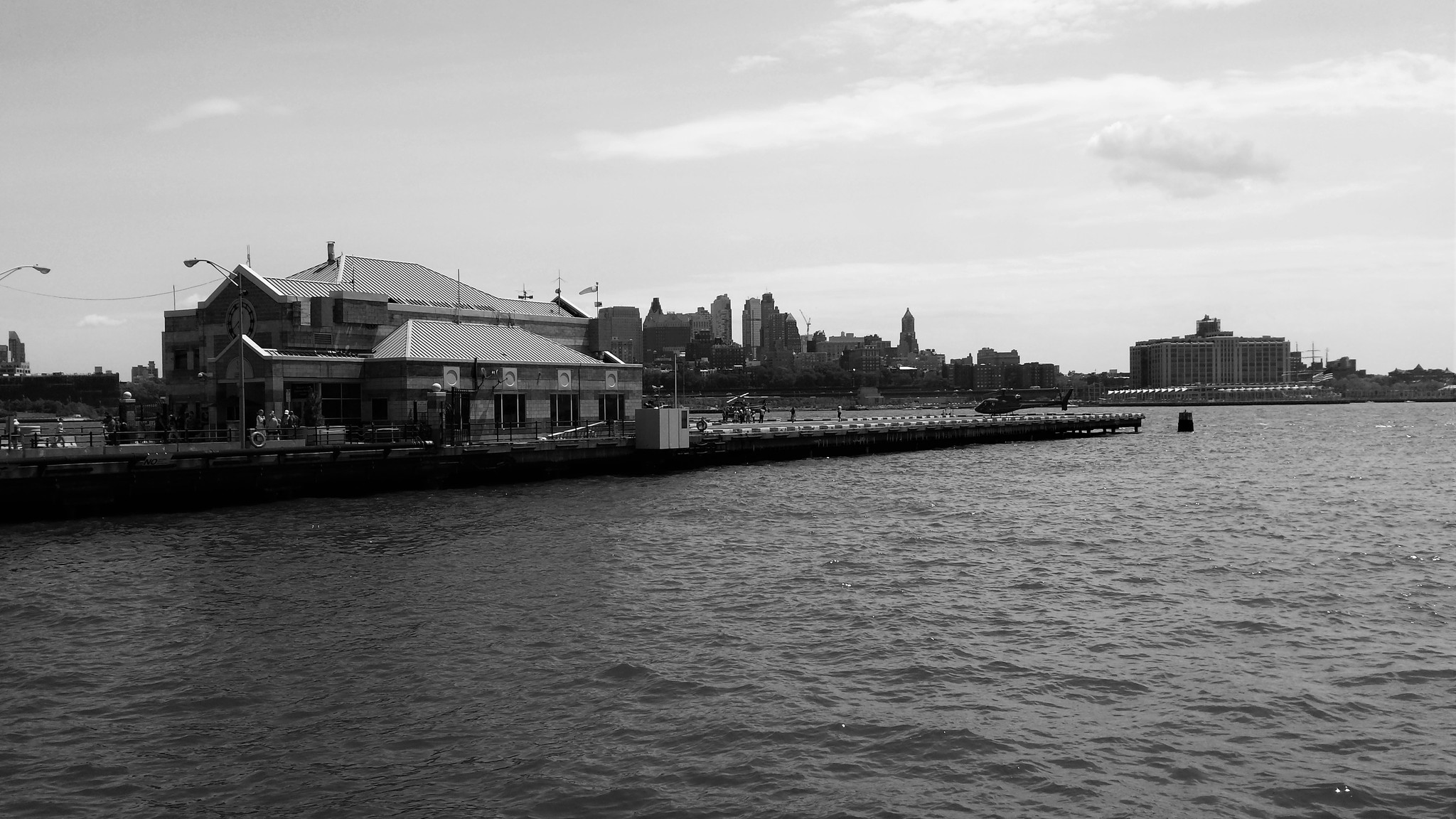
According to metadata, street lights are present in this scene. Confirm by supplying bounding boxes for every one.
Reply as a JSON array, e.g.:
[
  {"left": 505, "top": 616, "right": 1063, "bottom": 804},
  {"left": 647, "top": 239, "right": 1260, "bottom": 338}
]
[{"left": 183, "top": 257, "right": 245, "bottom": 451}]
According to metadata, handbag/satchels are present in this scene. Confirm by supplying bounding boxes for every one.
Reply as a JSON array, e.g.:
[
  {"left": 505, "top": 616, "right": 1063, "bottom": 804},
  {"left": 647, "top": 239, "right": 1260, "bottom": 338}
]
[{"left": 144, "top": 429, "right": 146, "bottom": 431}]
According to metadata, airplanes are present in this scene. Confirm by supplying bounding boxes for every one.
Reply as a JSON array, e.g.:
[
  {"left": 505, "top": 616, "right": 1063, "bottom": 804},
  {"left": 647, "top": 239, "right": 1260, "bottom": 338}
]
[{"left": 975, "top": 386, "right": 1074, "bottom": 419}]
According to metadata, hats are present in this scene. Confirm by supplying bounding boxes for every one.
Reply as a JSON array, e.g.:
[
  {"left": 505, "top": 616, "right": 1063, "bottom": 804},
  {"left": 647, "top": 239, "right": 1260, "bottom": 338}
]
[{"left": 284, "top": 410, "right": 289, "bottom": 415}]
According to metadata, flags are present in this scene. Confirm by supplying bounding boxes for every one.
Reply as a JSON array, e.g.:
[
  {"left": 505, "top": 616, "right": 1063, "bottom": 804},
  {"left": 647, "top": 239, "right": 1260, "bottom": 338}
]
[{"left": 1312, "top": 367, "right": 1333, "bottom": 383}]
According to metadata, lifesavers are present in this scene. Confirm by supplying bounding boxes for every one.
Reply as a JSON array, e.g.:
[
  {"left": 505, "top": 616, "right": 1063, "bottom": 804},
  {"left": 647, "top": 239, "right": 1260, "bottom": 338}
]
[
  {"left": 696, "top": 420, "right": 708, "bottom": 432},
  {"left": 250, "top": 432, "right": 266, "bottom": 448}
]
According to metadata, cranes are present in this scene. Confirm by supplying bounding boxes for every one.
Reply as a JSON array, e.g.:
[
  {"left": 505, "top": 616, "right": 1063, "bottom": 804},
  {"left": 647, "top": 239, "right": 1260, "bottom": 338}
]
[{"left": 799, "top": 309, "right": 811, "bottom": 341}]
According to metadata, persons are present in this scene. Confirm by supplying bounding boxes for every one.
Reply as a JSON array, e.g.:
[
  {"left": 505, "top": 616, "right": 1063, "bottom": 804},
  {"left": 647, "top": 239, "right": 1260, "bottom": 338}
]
[
  {"left": 768, "top": 407, "right": 770, "bottom": 412},
  {"left": 155, "top": 409, "right": 209, "bottom": 443},
  {"left": 101, "top": 413, "right": 131, "bottom": 446},
  {"left": 10, "top": 419, "right": 20, "bottom": 450},
  {"left": 137, "top": 417, "right": 149, "bottom": 443},
  {"left": 722, "top": 406, "right": 755, "bottom": 424},
  {"left": 50, "top": 418, "right": 68, "bottom": 448},
  {"left": 837, "top": 404, "right": 842, "bottom": 422},
  {"left": 757, "top": 408, "right": 765, "bottom": 423},
  {"left": 790, "top": 407, "right": 796, "bottom": 423},
  {"left": 267, "top": 410, "right": 299, "bottom": 440},
  {"left": 256, "top": 409, "right": 267, "bottom": 441}
]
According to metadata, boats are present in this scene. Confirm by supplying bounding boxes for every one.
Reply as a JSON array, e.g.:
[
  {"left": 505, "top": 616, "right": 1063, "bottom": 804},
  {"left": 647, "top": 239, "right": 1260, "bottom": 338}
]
[
  {"left": 901, "top": 408, "right": 912, "bottom": 410},
  {"left": 798, "top": 409, "right": 808, "bottom": 411},
  {"left": 916, "top": 408, "right": 922, "bottom": 410},
  {"left": 913, "top": 408, "right": 916, "bottom": 410},
  {"left": 931, "top": 406, "right": 939, "bottom": 409},
  {"left": 946, "top": 407, "right": 950, "bottom": 409},
  {"left": 951, "top": 405, "right": 958, "bottom": 409}
]
